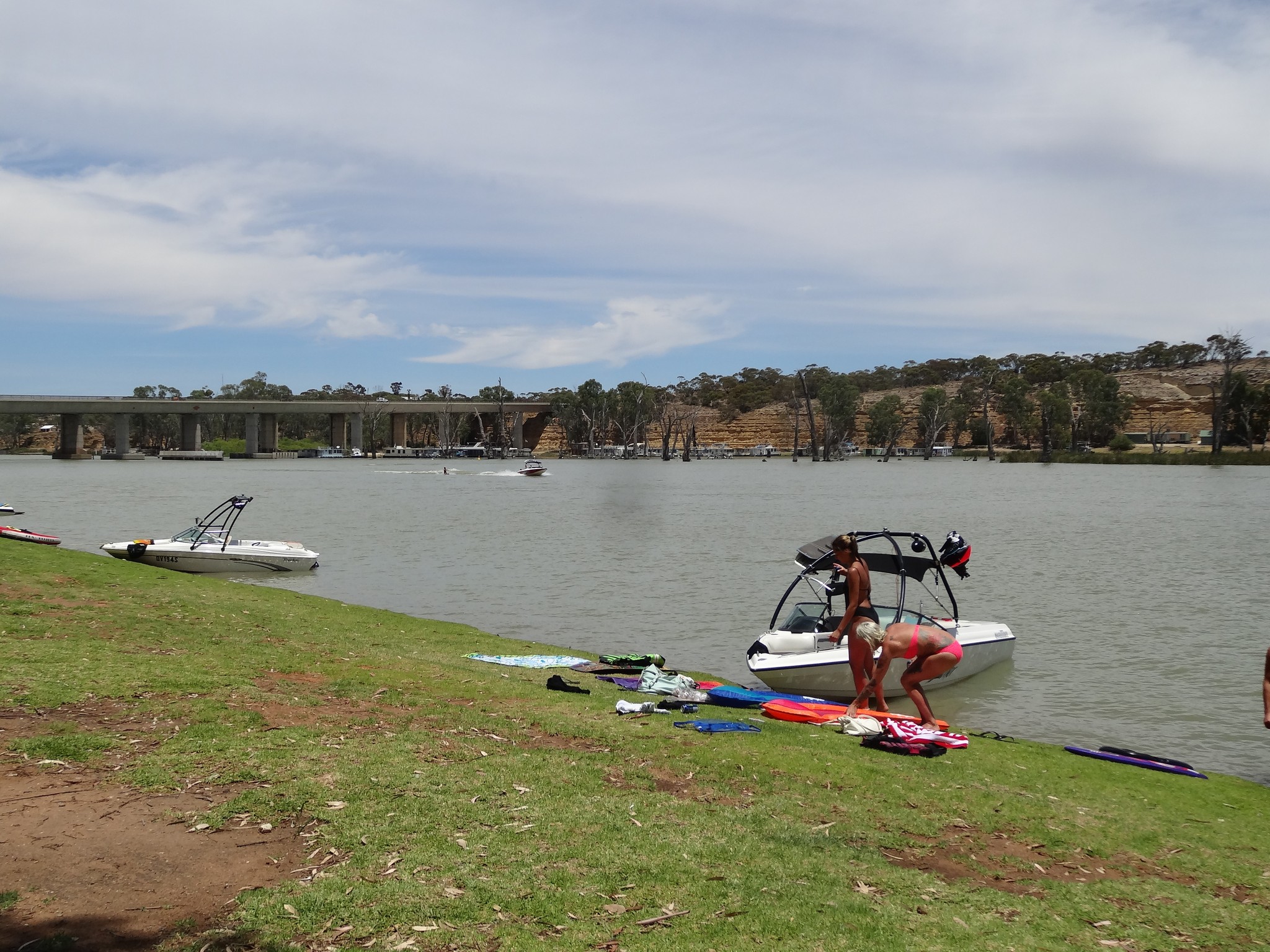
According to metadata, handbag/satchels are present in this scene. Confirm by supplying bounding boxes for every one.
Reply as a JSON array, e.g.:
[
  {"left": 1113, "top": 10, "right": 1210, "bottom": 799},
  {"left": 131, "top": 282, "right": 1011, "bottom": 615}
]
[
  {"left": 837, "top": 713, "right": 884, "bottom": 736},
  {"left": 599, "top": 653, "right": 665, "bottom": 668}
]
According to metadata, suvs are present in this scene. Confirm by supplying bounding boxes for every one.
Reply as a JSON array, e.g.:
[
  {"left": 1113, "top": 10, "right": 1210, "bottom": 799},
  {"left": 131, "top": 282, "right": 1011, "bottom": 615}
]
[{"left": 376, "top": 397, "right": 388, "bottom": 403}]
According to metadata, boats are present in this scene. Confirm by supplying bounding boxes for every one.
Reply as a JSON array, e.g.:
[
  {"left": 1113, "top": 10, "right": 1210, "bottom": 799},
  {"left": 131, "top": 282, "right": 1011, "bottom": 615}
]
[
  {"left": 745, "top": 527, "right": 1017, "bottom": 707},
  {"left": 350, "top": 446, "right": 365, "bottom": 458},
  {"left": 99, "top": 493, "right": 320, "bottom": 574},
  {"left": 0, "top": 525, "right": 62, "bottom": 546},
  {"left": 517, "top": 456, "right": 547, "bottom": 476},
  {"left": 0, "top": 503, "right": 26, "bottom": 517}
]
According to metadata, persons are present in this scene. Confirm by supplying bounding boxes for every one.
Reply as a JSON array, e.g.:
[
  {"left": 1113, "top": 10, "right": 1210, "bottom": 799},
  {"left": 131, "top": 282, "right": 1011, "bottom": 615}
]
[
  {"left": 844, "top": 621, "right": 963, "bottom": 731},
  {"left": 1263, "top": 647, "right": 1270, "bottom": 730},
  {"left": 828, "top": 535, "right": 889, "bottom": 712}
]
